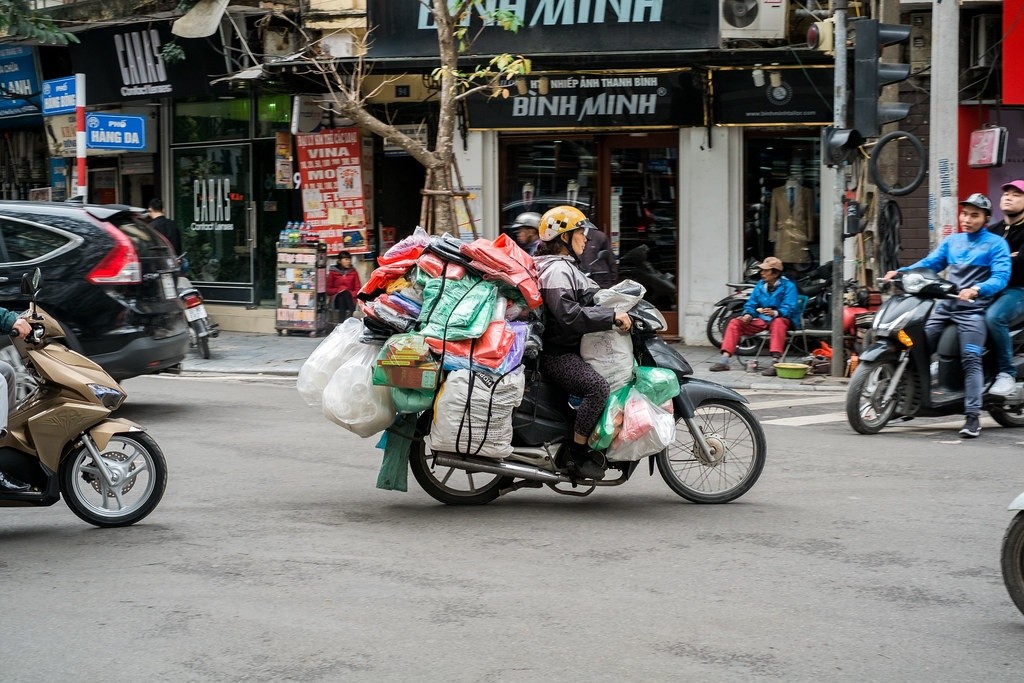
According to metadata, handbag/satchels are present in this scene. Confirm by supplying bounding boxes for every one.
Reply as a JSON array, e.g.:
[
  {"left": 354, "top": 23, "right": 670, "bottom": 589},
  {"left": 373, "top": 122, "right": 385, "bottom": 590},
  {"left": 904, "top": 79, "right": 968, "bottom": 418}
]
[
  {"left": 423, "top": 362, "right": 526, "bottom": 458},
  {"left": 586, "top": 365, "right": 681, "bottom": 464},
  {"left": 321, "top": 341, "right": 397, "bottom": 440},
  {"left": 578, "top": 279, "right": 648, "bottom": 392},
  {"left": 371, "top": 331, "right": 443, "bottom": 418},
  {"left": 294, "top": 316, "right": 369, "bottom": 414}
]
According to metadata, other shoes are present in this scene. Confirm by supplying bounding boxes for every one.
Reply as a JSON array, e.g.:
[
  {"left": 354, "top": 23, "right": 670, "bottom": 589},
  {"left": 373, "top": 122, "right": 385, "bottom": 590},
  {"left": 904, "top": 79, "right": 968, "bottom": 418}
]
[
  {"left": 709, "top": 362, "right": 730, "bottom": 371},
  {"left": 560, "top": 449, "right": 606, "bottom": 481},
  {"left": 761, "top": 365, "right": 776, "bottom": 376},
  {"left": 957, "top": 415, "right": 983, "bottom": 437}
]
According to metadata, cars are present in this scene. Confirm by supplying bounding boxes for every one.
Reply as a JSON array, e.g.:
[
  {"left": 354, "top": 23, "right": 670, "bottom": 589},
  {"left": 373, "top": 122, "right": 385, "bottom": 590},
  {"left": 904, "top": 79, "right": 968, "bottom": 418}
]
[{"left": 495, "top": 195, "right": 594, "bottom": 241}]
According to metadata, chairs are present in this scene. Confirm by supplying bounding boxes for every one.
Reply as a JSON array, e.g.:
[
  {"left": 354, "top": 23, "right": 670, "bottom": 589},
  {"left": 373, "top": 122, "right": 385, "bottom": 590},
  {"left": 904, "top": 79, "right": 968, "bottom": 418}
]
[{"left": 735, "top": 295, "right": 809, "bottom": 367}]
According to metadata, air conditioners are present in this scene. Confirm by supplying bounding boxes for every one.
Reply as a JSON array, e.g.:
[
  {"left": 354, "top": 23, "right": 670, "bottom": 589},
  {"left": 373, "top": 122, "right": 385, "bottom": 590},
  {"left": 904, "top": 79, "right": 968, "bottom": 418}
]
[
  {"left": 969, "top": 12, "right": 1002, "bottom": 68},
  {"left": 718, "top": 0, "right": 790, "bottom": 40}
]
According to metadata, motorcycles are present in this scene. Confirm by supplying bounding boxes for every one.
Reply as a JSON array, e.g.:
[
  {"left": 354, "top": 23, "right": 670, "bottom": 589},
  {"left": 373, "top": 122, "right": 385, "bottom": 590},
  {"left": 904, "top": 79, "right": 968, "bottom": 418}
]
[
  {"left": 1, "top": 269, "right": 168, "bottom": 527},
  {"left": 376, "top": 250, "right": 767, "bottom": 505},
  {"left": 845, "top": 267, "right": 1023, "bottom": 436},
  {"left": 176, "top": 251, "right": 220, "bottom": 358},
  {"left": 707, "top": 261, "right": 883, "bottom": 356}
]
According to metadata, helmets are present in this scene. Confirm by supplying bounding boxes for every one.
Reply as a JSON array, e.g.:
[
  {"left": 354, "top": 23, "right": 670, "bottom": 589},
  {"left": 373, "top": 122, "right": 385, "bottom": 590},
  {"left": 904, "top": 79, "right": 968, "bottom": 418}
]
[
  {"left": 510, "top": 211, "right": 543, "bottom": 234},
  {"left": 538, "top": 205, "right": 599, "bottom": 242}
]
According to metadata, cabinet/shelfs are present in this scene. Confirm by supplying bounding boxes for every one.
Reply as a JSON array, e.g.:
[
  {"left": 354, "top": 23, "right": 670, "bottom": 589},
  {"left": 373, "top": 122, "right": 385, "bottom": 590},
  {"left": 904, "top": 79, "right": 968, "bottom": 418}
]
[{"left": 275, "top": 243, "right": 328, "bottom": 338}]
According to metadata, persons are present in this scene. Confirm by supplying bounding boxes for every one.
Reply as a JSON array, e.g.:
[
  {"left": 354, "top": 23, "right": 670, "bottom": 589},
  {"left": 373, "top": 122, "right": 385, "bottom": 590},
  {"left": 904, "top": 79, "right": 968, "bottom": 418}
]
[
  {"left": 710, "top": 257, "right": 801, "bottom": 376},
  {"left": 145, "top": 196, "right": 183, "bottom": 271},
  {"left": 510, "top": 209, "right": 619, "bottom": 289},
  {"left": 326, "top": 251, "right": 361, "bottom": 323},
  {"left": 531, "top": 205, "right": 632, "bottom": 480},
  {"left": 986, "top": 180, "right": 1024, "bottom": 397},
  {"left": 0, "top": 306, "right": 33, "bottom": 493},
  {"left": 883, "top": 193, "right": 1012, "bottom": 439}
]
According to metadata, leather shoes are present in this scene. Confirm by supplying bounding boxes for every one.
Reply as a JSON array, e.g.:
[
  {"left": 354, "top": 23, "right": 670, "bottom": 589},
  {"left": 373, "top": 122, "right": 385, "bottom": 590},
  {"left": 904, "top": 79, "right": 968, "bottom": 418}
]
[{"left": 0, "top": 470, "right": 31, "bottom": 493}]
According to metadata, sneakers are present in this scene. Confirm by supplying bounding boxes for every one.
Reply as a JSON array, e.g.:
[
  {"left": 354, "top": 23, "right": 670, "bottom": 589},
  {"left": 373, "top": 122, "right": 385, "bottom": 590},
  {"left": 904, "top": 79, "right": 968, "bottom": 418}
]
[{"left": 987, "top": 372, "right": 1017, "bottom": 396}]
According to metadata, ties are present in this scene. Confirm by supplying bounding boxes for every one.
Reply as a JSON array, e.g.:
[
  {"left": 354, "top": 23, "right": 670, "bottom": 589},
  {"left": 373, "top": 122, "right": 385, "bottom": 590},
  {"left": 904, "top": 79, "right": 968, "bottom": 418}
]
[{"left": 789, "top": 187, "right": 795, "bottom": 214}]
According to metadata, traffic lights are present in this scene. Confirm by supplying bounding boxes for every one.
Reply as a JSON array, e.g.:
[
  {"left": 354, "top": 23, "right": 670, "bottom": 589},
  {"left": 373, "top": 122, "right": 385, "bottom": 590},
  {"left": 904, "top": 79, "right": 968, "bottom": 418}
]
[
  {"left": 820, "top": 127, "right": 863, "bottom": 166},
  {"left": 854, "top": 18, "right": 911, "bottom": 139},
  {"left": 807, "top": 21, "right": 833, "bottom": 52}
]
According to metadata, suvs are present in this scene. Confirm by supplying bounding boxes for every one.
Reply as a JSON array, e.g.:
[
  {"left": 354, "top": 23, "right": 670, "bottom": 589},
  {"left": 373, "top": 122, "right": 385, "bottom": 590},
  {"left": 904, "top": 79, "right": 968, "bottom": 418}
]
[{"left": 0, "top": 200, "right": 191, "bottom": 384}]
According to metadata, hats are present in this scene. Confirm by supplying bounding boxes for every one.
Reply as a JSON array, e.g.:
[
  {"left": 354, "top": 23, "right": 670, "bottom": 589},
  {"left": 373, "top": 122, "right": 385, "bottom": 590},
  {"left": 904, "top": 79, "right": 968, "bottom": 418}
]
[
  {"left": 958, "top": 193, "right": 993, "bottom": 218},
  {"left": 754, "top": 256, "right": 784, "bottom": 271},
  {"left": 1000, "top": 179, "right": 1024, "bottom": 193}
]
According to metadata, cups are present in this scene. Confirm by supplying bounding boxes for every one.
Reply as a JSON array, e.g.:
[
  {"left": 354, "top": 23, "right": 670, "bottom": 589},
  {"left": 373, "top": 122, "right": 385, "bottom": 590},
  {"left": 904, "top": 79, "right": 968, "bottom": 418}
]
[{"left": 324, "top": 364, "right": 395, "bottom": 437}]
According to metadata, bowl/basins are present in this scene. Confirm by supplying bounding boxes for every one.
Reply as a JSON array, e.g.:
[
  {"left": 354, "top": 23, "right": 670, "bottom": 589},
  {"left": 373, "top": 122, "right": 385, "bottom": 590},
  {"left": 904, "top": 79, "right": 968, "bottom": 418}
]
[{"left": 773, "top": 363, "right": 811, "bottom": 378}]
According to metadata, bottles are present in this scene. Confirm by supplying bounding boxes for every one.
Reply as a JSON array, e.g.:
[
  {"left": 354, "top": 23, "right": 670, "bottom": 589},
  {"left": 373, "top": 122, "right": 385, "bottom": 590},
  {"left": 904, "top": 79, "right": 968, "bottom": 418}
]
[{"left": 279, "top": 221, "right": 319, "bottom": 243}]
